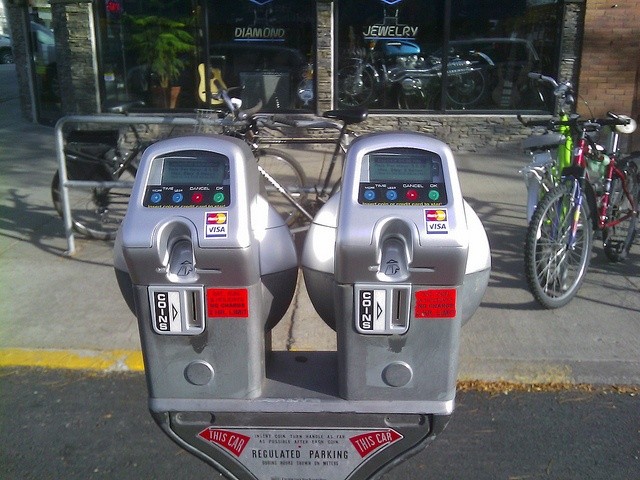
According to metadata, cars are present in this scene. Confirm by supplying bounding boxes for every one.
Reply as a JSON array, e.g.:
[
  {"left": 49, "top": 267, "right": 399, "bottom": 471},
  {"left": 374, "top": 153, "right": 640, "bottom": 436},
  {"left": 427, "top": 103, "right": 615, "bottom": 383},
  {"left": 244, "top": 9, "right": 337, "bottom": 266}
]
[{"left": 0, "top": 35, "right": 15, "bottom": 64}]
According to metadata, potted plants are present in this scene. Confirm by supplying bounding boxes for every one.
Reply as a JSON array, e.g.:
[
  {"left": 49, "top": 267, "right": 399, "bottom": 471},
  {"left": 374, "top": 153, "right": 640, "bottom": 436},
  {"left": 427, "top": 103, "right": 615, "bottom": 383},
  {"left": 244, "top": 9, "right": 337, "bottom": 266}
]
[{"left": 128, "top": 14, "right": 202, "bottom": 112}]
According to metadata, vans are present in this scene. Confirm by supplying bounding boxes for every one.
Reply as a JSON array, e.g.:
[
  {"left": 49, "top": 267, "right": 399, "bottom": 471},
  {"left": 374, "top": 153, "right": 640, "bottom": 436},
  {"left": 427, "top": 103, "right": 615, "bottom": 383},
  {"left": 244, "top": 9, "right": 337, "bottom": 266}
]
[
  {"left": 432, "top": 37, "right": 542, "bottom": 110},
  {"left": 178, "top": 44, "right": 308, "bottom": 109}
]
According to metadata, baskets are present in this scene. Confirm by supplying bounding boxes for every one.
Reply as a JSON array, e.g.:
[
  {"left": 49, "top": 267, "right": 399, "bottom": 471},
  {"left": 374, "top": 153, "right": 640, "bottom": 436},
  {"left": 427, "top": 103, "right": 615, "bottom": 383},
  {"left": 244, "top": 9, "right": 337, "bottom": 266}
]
[{"left": 195, "top": 108, "right": 225, "bottom": 134}]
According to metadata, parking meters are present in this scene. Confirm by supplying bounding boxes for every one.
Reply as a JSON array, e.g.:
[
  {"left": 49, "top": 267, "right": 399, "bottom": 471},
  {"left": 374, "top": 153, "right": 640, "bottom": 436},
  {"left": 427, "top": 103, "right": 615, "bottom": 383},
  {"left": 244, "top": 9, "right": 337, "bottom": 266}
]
[{"left": 113, "top": 131, "right": 493, "bottom": 479}]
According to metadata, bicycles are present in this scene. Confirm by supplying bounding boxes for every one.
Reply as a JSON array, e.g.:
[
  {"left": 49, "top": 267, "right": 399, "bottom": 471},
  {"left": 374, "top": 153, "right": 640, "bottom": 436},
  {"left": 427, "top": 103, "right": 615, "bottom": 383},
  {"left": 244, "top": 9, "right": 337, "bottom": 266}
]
[
  {"left": 202, "top": 85, "right": 368, "bottom": 233},
  {"left": 519, "top": 72, "right": 593, "bottom": 290},
  {"left": 52, "top": 98, "right": 306, "bottom": 241},
  {"left": 517, "top": 111, "right": 640, "bottom": 308}
]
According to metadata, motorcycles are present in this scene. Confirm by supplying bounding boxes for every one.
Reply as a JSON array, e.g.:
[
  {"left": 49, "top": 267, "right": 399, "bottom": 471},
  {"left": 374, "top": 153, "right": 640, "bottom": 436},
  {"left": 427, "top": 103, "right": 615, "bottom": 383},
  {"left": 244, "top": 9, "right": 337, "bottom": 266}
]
[{"left": 338, "top": 37, "right": 494, "bottom": 109}]
